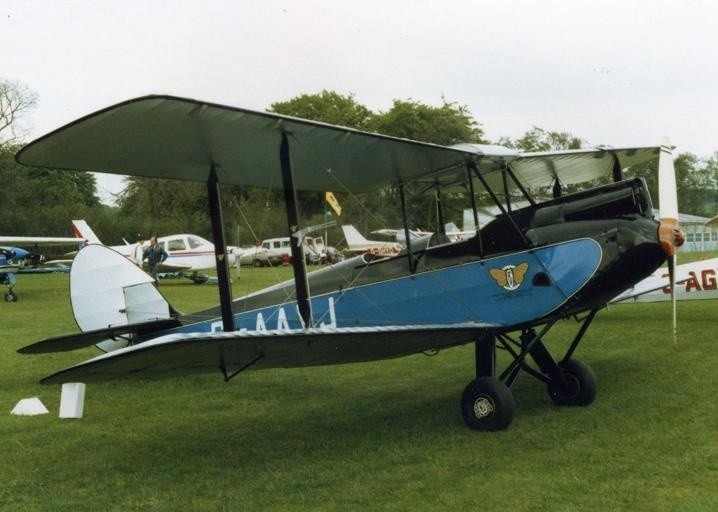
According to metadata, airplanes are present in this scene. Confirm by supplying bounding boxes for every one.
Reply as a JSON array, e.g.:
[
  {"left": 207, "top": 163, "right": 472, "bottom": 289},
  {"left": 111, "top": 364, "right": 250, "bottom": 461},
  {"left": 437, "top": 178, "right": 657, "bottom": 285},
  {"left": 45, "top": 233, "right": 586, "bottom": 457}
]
[{"left": 5, "top": 94, "right": 690, "bottom": 420}]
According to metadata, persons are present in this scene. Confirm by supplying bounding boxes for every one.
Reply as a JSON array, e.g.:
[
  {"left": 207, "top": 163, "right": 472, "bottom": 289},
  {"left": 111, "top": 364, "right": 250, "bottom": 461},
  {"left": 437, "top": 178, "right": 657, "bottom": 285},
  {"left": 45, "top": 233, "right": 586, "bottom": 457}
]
[
  {"left": 31, "top": 243, "right": 45, "bottom": 268},
  {"left": 134, "top": 236, "right": 168, "bottom": 287}
]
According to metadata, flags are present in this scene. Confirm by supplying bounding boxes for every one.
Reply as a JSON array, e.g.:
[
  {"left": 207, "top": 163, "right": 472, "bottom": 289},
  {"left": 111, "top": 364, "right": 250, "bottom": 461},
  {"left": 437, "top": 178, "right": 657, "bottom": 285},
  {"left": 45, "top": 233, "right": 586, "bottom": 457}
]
[{"left": 326, "top": 191, "right": 342, "bottom": 216}]
